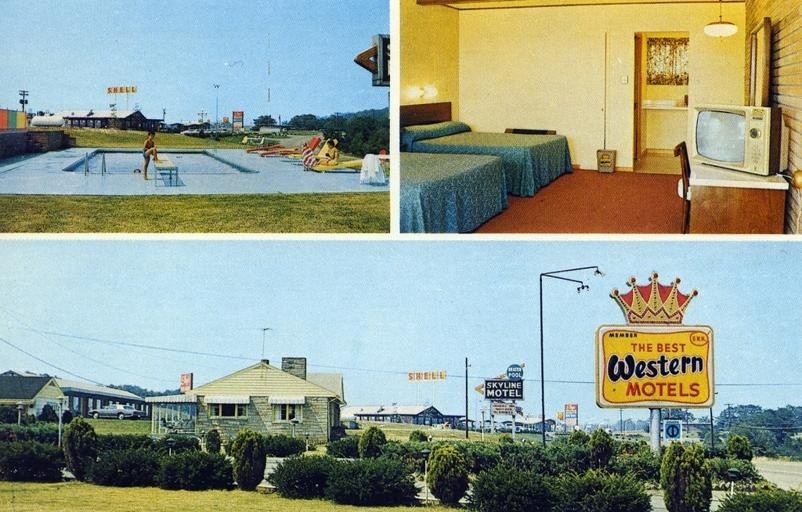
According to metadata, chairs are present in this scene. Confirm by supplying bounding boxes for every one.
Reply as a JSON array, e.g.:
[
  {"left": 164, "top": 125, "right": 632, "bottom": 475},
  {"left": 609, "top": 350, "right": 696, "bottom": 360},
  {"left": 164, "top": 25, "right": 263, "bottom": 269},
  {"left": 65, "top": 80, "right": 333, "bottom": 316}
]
[
  {"left": 673, "top": 141, "right": 691, "bottom": 234},
  {"left": 245, "top": 136, "right": 365, "bottom": 174}
]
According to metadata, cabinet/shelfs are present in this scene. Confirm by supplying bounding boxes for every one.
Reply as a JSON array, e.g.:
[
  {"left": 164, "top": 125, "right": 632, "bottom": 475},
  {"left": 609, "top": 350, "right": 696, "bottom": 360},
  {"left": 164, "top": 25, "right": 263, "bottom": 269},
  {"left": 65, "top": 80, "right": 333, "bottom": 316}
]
[{"left": 689, "top": 162, "right": 790, "bottom": 235}]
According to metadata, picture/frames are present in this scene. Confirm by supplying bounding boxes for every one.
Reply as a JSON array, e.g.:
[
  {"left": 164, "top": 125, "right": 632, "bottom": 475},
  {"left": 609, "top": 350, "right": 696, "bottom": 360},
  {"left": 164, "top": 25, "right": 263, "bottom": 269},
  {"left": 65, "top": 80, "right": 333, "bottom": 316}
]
[{"left": 749, "top": 17, "right": 771, "bottom": 106}]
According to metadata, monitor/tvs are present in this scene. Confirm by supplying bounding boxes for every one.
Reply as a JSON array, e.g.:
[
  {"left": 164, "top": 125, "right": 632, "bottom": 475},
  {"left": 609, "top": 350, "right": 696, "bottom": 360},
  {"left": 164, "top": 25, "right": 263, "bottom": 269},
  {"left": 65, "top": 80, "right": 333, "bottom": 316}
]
[{"left": 689, "top": 105, "right": 789, "bottom": 178}]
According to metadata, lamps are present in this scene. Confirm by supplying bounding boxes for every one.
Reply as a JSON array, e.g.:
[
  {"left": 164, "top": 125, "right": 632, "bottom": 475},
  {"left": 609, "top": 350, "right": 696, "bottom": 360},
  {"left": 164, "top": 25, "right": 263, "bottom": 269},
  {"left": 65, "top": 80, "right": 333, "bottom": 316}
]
[{"left": 703, "top": 0, "right": 739, "bottom": 38}]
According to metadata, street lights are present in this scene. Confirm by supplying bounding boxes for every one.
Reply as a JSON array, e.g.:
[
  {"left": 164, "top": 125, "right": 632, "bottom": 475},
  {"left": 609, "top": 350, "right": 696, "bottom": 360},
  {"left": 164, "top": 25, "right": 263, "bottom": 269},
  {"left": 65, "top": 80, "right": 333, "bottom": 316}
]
[
  {"left": 15, "top": 399, "right": 25, "bottom": 426},
  {"left": 727, "top": 467, "right": 742, "bottom": 498},
  {"left": 421, "top": 448, "right": 431, "bottom": 509},
  {"left": 213, "top": 83, "right": 221, "bottom": 124},
  {"left": 55, "top": 393, "right": 64, "bottom": 448},
  {"left": 536, "top": 264, "right": 605, "bottom": 448}
]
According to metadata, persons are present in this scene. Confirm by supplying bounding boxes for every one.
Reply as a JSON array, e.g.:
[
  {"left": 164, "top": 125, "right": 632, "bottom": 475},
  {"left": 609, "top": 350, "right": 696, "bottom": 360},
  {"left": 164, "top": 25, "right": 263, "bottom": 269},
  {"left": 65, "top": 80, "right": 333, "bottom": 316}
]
[
  {"left": 142, "top": 131, "right": 162, "bottom": 181},
  {"left": 317, "top": 140, "right": 340, "bottom": 165}
]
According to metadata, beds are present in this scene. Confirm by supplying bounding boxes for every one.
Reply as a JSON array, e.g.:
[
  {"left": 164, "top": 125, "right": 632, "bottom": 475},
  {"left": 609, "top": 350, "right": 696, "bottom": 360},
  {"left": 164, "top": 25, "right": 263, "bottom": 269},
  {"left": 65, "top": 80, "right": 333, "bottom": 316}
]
[
  {"left": 400, "top": 150, "right": 511, "bottom": 233},
  {"left": 400, "top": 101, "right": 575, "bottom": 199}
]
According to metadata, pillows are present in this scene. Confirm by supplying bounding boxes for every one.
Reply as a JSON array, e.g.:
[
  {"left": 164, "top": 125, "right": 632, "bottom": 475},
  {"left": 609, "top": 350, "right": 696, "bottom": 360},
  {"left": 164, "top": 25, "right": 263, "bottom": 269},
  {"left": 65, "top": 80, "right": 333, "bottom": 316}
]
[{"left": 401, "top": 120, "right": 471, "bottom": 144}]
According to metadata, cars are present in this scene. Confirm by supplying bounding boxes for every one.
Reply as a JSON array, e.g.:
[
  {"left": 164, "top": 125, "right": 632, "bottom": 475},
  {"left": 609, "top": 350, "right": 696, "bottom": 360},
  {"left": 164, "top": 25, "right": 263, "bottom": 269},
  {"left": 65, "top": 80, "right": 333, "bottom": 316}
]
[{"left": 87, "top": 403, "right": 144, "bottom": 421}]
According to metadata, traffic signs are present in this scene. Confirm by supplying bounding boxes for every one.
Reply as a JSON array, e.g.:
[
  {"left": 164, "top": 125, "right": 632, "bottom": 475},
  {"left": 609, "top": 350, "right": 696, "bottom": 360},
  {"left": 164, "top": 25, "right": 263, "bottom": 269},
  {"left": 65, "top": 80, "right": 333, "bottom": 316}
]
[{"left": 351, "top": 31, "right": 390, "bottom": 88}]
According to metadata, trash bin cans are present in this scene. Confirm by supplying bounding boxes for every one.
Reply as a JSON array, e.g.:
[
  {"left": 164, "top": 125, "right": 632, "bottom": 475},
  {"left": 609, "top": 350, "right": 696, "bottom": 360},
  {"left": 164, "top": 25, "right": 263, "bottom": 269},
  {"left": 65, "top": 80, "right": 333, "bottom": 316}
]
[{"left": 595, "top": 149, "right": 617, "bottom": 176}]
